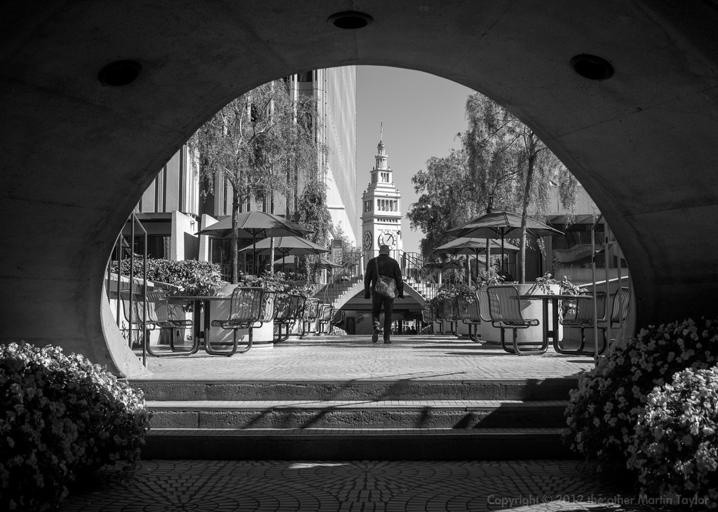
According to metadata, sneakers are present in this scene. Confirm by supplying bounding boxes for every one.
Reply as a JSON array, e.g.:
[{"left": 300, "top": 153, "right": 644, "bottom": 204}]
[{"left": 371, "top": 324, "right": 393, "bottom": 344}]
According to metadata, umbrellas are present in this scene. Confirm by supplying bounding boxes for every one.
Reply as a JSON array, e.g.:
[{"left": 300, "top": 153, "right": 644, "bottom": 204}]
[
  {"left": 237, "top": 234, "right": 329, "bottom": 275},
  {"left": 432, "top": 236, "right": 519, "bottom": 278},
  {"left": 267, "top": 254, "right": 341, "bottom": 277},
  {"left": 192, "top": 207, "right": 311, "bottom": 278},
  {"left": 445, "top": 208, "right": 564, "bottom": 276},
  {"left": 422, "top": 254, "right": 492, "bottom": 277}
]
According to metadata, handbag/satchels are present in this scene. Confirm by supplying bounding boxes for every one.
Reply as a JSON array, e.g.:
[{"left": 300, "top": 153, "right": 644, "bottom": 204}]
[{"left": 374, "top": 273, "right": 399, "bottom": 299}]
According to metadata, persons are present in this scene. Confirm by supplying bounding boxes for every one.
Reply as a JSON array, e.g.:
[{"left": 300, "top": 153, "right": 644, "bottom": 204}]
[
  {"left": 363, "top": 245, "right": 404, "bottom": 343},
  {"left": 402, "top": 324, "right": 417, "bottom": 334}
]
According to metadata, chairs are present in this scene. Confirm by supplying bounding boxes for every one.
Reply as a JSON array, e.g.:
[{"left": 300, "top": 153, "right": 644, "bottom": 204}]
[
  {"left": 120, "top": 280, "right": 346, "bottom": 355},
  {"left": 421, "top": 284, "right": 630, "bottom": 357}
]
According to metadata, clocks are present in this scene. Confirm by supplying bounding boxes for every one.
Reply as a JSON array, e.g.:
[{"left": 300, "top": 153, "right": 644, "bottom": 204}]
[
  {"left": 379, "top": 231, "right": 396, "bottom": 251},
  {"left": 362, "top": 231, "right": 372, "bottom": 251}
]
